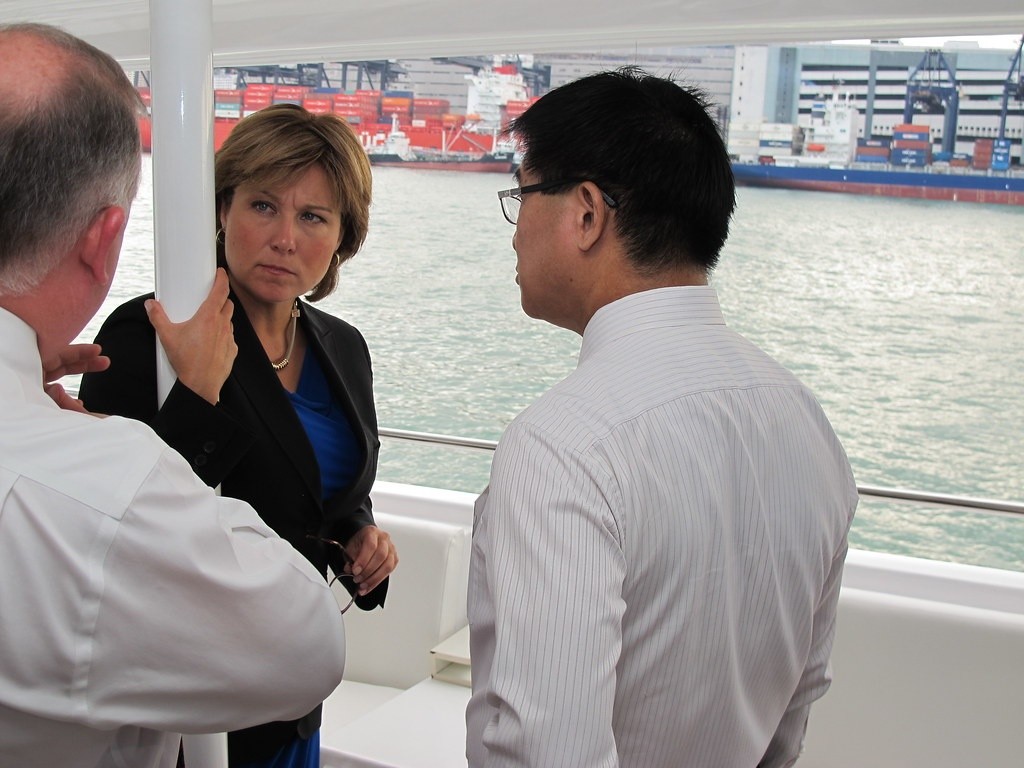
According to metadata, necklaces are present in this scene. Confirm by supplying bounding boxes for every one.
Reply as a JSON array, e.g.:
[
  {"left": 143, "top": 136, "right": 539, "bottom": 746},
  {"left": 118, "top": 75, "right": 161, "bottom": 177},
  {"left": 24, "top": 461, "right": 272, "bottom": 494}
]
[{"left": 269, "top": 299, "right": 301, "bottom": 370}]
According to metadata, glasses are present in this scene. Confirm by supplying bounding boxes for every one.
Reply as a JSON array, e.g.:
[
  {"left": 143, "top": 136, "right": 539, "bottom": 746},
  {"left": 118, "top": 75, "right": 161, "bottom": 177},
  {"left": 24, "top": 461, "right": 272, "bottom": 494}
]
[
  {"left": 304, "top": 534, "right": 363, "bottom": 615},
  {"left": 498, "top": 177, "right": 617, "bottom": 225}
]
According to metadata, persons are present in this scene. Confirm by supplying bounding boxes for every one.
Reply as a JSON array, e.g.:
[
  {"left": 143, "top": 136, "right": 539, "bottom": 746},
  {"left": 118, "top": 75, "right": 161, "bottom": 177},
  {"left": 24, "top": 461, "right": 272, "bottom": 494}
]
[
  {"left": 0, "top": 21, "right": 348, "bottom": 768},
  {"left": 74, "top": 101, "right": 401, "bottom": 766},
  {"left": 464, "top": 66, "right": 861, "bottom": 768}
]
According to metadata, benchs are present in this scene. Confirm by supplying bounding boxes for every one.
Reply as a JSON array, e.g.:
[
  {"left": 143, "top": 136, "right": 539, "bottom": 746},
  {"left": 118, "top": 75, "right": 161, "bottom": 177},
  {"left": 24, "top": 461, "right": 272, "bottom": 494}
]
[{"left": 320, "top": 478, "right": 1024, "bottom": 768}]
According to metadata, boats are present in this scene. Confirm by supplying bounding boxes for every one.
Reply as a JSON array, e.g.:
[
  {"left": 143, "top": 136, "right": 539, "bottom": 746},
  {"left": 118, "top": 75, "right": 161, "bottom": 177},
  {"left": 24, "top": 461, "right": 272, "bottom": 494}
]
[
  {"left": 364, "top": 147, "right": 516, "bottom": 174},
  {"left": 729, "top": 89, "right": 1024, "bottom": 206}
]
